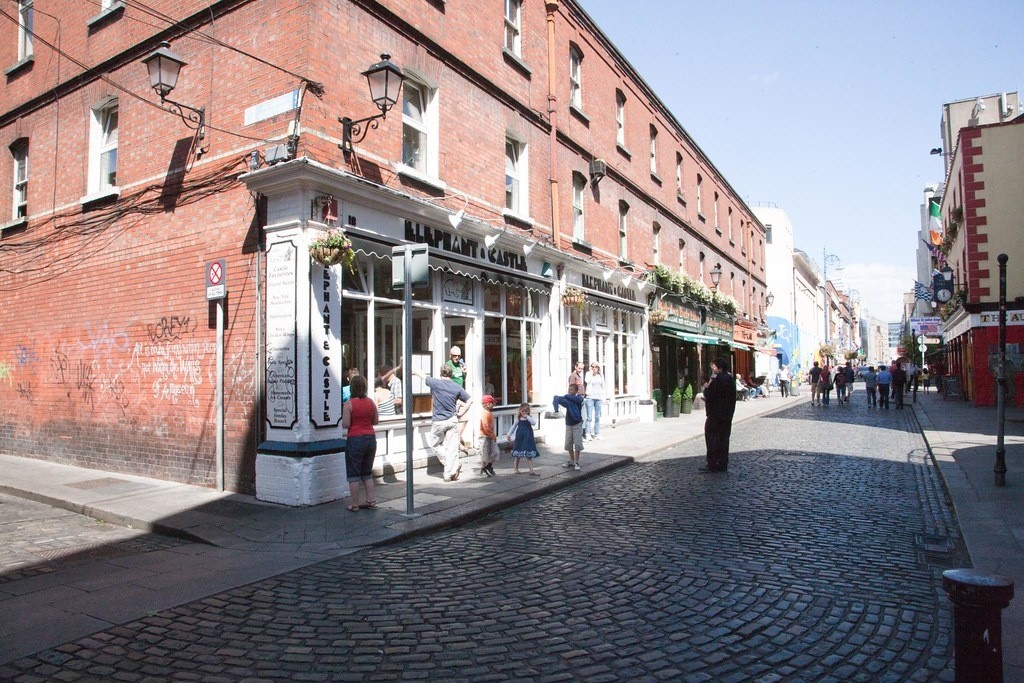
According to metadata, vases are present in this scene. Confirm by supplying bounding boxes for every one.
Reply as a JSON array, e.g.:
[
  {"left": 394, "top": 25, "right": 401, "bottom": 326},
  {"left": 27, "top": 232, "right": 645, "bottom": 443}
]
[
  {"left": 310, "top": 245, "right": 346, "bottom": 265},
  {"left": 562, "top": 296, "right": 583, "bottom": 307}
]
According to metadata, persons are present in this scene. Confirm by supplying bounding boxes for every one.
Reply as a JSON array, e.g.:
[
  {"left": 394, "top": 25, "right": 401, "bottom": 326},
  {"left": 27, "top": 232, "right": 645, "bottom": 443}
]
[
  {"left": 698, "top": 357, "right": 735, "bottom": 470},
  {"left": 342, "top": 376, "right": 379, "bottom": 512},
  {"left": 777, "top": 365, "right": 789, "bottom": 398},
  {"left": 865, "top": 359, "right": 948, "bottom": 409},
  {"left": 342, "top": 356, "right": 402, "bottom": 416},
  {"left": 585, "top": 361, "right": 606, "bottom": 441},
  {"left": 485, "top": 376, "right": 494, "bottom": 395},
  {"left": 553, "top": 384, "right": 584, "bottom": 470},
  {"left": 808, "top": 361, "right": 859, "bottom": 407},
  {"left": 479, "top": 396, "right": 500, "bottom": 477},
  {"left": 701, "top": 360, "right": 733, "bottom": 402},
  {"left": 506, "top": 403, "right": 541, "bottom": 476},
  {"left": 736, "top": 372, "right": 770, "bottom": 402},
  {"left": 568, "top": 361, "right": 586, "bottom": 443},
  {"left": 412, "top": 365, "right": 473, "bottom": 482},
  {"left": 445, "top": 345, "right": 467, "bottom": 386}
]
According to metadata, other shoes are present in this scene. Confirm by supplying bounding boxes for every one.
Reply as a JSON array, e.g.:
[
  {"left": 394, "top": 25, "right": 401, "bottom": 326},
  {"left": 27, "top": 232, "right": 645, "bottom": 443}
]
[
  {"left": 444, "top": 477, "right": 451, "bottom": 482},
  {"left": 717, "top": 464, "right": 728, "bottom": 472},
  {"left": 561, "top": 461, "right": 574, "bottom": 468},
  {"left": 697, "top": 465, "right": 711, "bottom": 473},
  {"left": 574, "top": 461, "right": 581, "bottom": 470},
  {"left": 480, "top": 468, "right": 488, "bottom": 477},
  {"left": 529, "top": 471, "right": 540, "bottom": 477},
  {"left": 585, "top": 432, "right": 594, "bottom": 441},
  {"left": 595, "top": 435, "right": 603, "bottom": 441},
  {"left": 581, "top": 436, "right": 589, "bottom": 442},
  {"left": 484, "top": 466, "right": 495, "bottom": 477},
  {"left": 451, "top": 464, "right": 462, "bottom": 481},
  {"left": 514, "top": 468, "right": 521, "bottom": 474}
]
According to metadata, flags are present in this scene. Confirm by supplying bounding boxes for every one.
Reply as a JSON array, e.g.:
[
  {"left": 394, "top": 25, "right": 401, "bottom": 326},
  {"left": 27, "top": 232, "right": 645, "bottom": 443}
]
[
  {"left": 915, "top": 280, "right": 932, "bottom": 303},
  {"left": 930, "top": 269, "right": 942, "bottom": 289},
  {"left": 923, "top": 240, "right": 946, "bottom": 266}
]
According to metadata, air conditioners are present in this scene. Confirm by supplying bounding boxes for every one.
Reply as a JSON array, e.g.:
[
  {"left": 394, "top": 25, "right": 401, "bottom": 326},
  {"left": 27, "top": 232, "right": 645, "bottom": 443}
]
[{"left": 589, "top": 160, "right": 606, "bottom": 188}]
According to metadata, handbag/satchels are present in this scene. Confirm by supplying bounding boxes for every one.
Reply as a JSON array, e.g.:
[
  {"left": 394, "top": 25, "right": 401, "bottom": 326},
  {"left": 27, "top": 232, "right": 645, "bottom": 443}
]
[{"left": 828, "top": 383, "right": 834, "bottom": 391}]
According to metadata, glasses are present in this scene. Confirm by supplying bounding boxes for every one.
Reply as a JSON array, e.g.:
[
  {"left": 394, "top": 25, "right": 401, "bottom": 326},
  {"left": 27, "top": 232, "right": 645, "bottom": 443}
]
[
  {"left": 520, "top": 410, "right": 529, "bottom": 413},
  {"left": 591, "top": 366, "right": 599, "bottom": 369}
]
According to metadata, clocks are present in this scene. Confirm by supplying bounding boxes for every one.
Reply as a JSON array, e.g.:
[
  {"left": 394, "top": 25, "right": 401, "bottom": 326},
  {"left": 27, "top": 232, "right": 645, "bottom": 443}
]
[{"left": 936, "top": 288, "right": 951, "bottom": 302}]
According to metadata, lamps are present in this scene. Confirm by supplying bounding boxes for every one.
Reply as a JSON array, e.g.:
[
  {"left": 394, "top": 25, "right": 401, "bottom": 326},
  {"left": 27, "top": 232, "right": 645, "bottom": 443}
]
[
  {"left": 709, "top": 265, "right": 723, "bottom": 289},
  {"left": 760, "top": 292, "right": 774, "bottom": 311},
  {"left": 931, "top": 148, "right": 954, "bottom": 156},
  {"left": 423, "top": 193, "right": 469, "bottom": 229},
  {"left": 596, "top": 259, "right": 651, "bottom": 291},
  {"left": 478, "top": 217, "right": 507, "bottom": 249},
  {"left": 338, "top": 52, "right": 410, "bottom": 163},
  {"left": 942, "top": 261, "right": 968, "bottom": 292},
  {"left": 141, "top": 40, "right": 205, "bottom": 140},
  {"left": 513, "top": 228, "right": 542, "bottom": 256}
]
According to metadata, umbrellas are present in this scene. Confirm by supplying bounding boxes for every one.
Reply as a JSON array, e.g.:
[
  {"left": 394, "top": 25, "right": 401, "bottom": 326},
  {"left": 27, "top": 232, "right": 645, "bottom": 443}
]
[{"left": 895, "top": 357, "right": 909, "bottom": 363}]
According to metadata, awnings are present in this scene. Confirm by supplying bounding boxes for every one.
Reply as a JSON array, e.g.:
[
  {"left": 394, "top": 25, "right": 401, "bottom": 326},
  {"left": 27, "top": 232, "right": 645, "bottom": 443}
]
[{"left": 662, "top": 328, "right": 777, "bottom": 356}]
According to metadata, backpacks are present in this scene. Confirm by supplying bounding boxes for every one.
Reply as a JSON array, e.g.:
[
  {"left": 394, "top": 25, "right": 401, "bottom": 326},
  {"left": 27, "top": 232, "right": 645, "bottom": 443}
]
[{"left": 837, "top": 372, "right": 846, "bottom": 386}]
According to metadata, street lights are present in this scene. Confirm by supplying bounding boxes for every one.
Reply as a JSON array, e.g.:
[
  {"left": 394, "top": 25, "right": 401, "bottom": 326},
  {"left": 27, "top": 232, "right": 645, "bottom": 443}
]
[
  {"left": 823, "top": 246, "right": 846, "bottom": 376},
  {"left": 859, "top": 307, "right": 871, "bottom": 366},
  {"left": 849, "top": 289, "right": 863, "bottom": 366}
]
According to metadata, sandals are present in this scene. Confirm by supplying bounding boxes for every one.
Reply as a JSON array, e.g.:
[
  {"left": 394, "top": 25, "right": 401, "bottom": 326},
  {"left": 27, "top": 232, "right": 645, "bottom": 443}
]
[
  {"left": 346, "top": 504, "right": 359, "bottom": 513},
  {"left": 359, "top": 499, "right": 377, "bottom": 510}
]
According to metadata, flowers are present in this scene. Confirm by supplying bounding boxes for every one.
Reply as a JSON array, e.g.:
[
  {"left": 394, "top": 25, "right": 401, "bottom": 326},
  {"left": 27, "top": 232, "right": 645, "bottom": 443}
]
[
  {"left": 308, "top": 229, "right": 355, "bottom": 275},
  {"left": 561, "top": 288, "right": 589, "bottom": 313}
]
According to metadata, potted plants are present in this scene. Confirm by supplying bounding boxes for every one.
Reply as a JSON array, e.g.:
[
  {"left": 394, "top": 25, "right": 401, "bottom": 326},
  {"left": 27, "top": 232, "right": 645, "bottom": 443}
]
[
  {"left": 672, "top": 387, "right": 682, "bottom": 417},
  {"left": 682, "top": 384, "right": 693, "bottom": 414}
]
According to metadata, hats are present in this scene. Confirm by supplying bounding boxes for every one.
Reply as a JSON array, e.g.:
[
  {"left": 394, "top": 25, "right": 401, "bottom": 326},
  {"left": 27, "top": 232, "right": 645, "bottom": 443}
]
[
  {"left": 481, "top": 395, "right": 498, "bottom": 404},
  {"left": 449, "top": 346, "right": 461, "bottom": 356}
]
